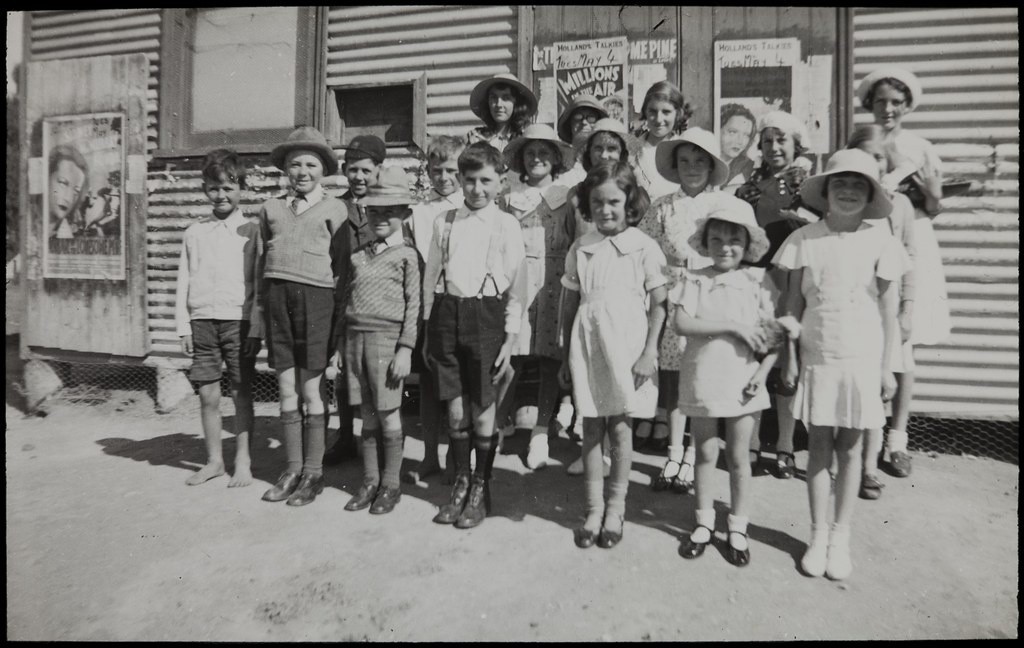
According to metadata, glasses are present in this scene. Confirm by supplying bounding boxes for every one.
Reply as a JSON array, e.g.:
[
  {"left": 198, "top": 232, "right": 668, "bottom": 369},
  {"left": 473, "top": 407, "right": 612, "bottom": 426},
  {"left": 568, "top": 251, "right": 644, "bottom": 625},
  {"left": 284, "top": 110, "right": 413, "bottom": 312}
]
[{"left": 570, "top": 113, "right": 598, "bottom": 123}]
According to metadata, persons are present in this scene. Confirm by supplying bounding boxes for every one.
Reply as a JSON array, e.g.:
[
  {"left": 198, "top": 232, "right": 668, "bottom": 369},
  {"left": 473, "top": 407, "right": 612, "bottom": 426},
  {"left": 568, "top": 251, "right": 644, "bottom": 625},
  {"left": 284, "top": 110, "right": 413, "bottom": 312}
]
[
  {"left": 668, "top": 195, "right": 779, "bottom": 566},
  {"left": 49, "top": 143, "right": 121, "bottom": 238},
  {"left": 324, "top": 69, "right": 950, "bottom": 489},
  {"left": 555, "top": 159, "right": 668, "bottom": 546},
  {"left": 175, "top": 149, "right": 263, "bottom": 489},
  {"left": 331, "top": 161, "right": 420, "bottom": 515},
  {"left": 422, "top": 141, "right": 526, "bottom": 524},
  {"left": 250, "top": 127, "right": 348, "bottom": 505},
  {"left": 771, "top": 149, "right": 913, "bottom": 579}
]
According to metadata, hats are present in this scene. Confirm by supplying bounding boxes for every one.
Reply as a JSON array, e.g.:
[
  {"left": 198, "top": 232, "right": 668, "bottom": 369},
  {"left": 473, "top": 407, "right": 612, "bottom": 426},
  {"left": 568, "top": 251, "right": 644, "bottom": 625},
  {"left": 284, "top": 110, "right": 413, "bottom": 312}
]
[
  {"left": 655, "top": 126, "right": 729, "bottom": 187},
  {"left": 687, "top": 203, "right": 771, "bottom": 263},
  {"left": 571, "top": 117, "right": 644, "bottom": 161},
  {"left": 502, "top": 123, "right": 578, "bottom": 175},
  {"left": 271, "top": 126, "right": 338, "bottom": 177},
  {"left": 358, "top": 163, "right": 418, "bottom": 206},
  {"left": 557, "top": 94, "right": 610, "bottom": 144},
  {"left": 470, "top": 72, "right": 538, "bottom": 120},
  {"left": 798, "top": 148, "right": 894, "bottom": 220},
  {"left": 858, "top": 64, "right": 922, "bottom": 114},
  {"left": 346, "top": 134, "right": 387, "bottom": 163},
  {"left": 756, "top": 110, "right": 810, "bottom": 151}
]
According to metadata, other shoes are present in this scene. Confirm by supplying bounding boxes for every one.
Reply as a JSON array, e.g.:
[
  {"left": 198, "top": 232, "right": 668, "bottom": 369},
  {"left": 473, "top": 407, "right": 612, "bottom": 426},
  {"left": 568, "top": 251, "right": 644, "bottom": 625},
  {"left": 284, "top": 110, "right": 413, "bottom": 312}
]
[
  {"left": 800, "top": 529, "right": 852, "bottom": 579},
  {"left": 577, "top": 512, "right": 624, "bottom": 548},
  {"left": 528, "top": 425, "right": 551, "bottom": 470},
  {"left": 633, "top": 422, "right": 912, "bottom": 500},
  {"left": 679, "top": 539, "right": 751, "bottom": 566}
]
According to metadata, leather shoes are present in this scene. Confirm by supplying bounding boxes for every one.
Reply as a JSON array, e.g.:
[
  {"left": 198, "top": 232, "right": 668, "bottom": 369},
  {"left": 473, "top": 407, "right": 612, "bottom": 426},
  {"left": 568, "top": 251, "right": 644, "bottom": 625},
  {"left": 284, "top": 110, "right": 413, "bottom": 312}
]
[
  {"left": 344, "top": 483, "right": 402, "bottom": 512},
  {"left": 264, "top": 470, "right": 325, "bottom": 507},
  {"left": 433, "top": 476, "right": 492, "bottom": 528}
]
